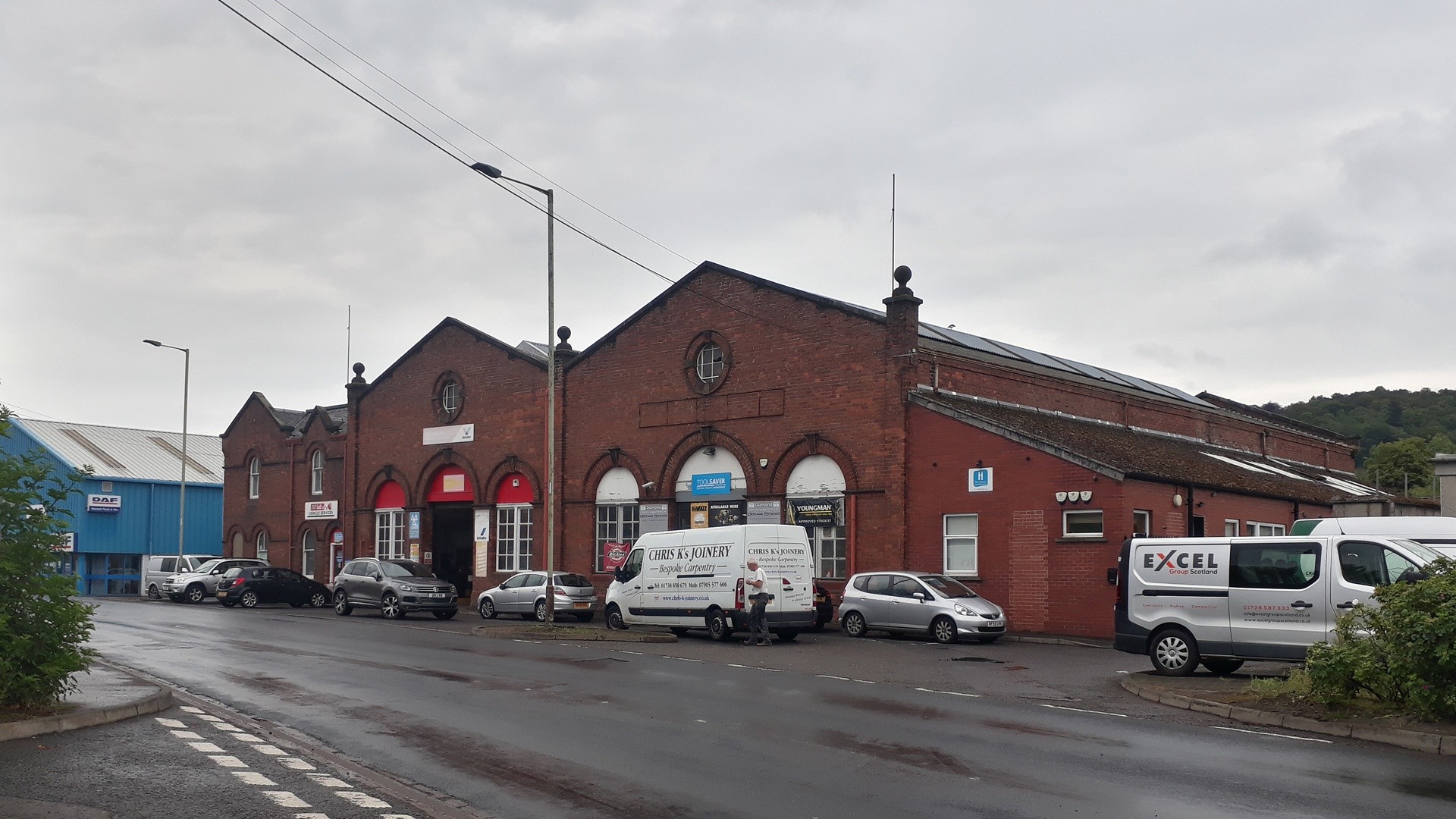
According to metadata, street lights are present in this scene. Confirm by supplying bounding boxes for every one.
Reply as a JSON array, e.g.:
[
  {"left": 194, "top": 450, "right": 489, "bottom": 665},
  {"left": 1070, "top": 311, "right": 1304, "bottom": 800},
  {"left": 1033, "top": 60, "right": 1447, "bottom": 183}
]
[
  {"left": 143, "top": 340, "right": 189, "bottom": 572},
  {"left": 472, "top": 162, "right": 554, "bottom": 625}
]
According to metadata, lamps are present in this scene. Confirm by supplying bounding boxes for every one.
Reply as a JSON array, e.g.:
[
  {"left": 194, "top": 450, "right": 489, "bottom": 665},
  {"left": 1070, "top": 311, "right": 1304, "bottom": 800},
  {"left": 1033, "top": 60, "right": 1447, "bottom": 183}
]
[
  {"left": 1199, "top": 502, "right": 1205, "bottom": 506},
  {"left": 703, "top": 448, "right": 716, "bottom": 455}
]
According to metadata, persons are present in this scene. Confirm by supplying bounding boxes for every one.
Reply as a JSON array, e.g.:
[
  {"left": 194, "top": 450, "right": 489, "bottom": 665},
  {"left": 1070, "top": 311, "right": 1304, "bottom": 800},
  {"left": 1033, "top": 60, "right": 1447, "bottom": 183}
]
[
  {"left": 1238, "top": 557, "right": 1304, "bottom": 588},
  {"left": 743, "top": 558, "right": 772, "bottom": 646}
]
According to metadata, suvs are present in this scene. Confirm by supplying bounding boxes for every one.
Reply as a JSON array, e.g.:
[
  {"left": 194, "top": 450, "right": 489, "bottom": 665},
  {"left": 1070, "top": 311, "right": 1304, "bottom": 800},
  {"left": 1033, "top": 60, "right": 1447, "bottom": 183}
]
[
  {"left": 161, "top": 558, "right": 271, "bottom": 604},
  {"left": 332, "top": 557, "right": 459, "bottom": 620}
]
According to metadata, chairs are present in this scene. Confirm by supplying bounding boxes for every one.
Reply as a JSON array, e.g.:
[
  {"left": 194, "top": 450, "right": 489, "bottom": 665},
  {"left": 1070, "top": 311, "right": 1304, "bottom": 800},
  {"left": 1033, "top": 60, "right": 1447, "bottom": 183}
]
[
  {"left": 896, "top": 583, "right": 909, "bottom": 596},
  {"left": 1231, "top": 558, "right": 1304, "bottom": 587}
]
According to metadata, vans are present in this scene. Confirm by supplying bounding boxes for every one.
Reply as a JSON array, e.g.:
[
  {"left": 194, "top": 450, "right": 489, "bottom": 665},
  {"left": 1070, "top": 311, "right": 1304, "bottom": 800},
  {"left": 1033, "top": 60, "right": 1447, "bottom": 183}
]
[
  {"left": 604, "top": 523, "right": 817, "bottom": 641},
  {"left": 1113, "top": 534, "right": 1456, "bottom": 677},
  {"left": 144, "top": 555, "right": 217, "bottom": 601},
  {"left": 1288, "top": 517, "right": 1456, "bottom": 561}
]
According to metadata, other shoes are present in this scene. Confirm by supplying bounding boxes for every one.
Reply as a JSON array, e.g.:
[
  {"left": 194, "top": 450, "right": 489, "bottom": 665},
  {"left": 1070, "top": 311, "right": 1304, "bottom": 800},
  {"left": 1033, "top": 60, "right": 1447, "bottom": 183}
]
[{"left": 744, "top": 640, "right": 772, "bottom": 646}]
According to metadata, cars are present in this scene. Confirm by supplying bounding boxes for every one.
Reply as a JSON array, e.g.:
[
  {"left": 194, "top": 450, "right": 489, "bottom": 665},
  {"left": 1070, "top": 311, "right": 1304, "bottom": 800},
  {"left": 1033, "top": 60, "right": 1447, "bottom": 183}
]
[
  {"left": 477, "top": 571, "right": 598, "bottom": 623},
  {"left": 215, "top": 566, "right": 332, "bottom": 608},
  {"left": 813, "top": 582, "right": 833, "bottom": 632},
  {"left": 837, "top": 571, "right": 1008, "bottom": 644}
]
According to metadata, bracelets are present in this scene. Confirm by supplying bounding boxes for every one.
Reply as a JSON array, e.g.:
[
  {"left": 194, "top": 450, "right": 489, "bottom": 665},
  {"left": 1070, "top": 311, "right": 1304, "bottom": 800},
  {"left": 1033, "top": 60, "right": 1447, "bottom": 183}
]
[{"left": 749, "top": 581, "right": 751, "bottom": 585}]
